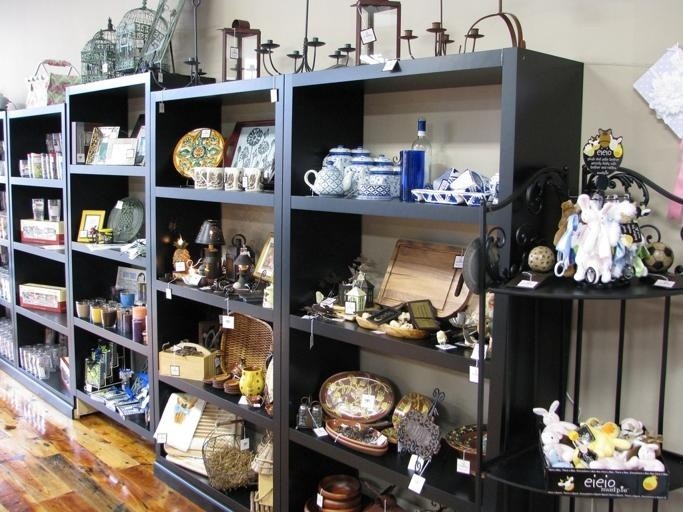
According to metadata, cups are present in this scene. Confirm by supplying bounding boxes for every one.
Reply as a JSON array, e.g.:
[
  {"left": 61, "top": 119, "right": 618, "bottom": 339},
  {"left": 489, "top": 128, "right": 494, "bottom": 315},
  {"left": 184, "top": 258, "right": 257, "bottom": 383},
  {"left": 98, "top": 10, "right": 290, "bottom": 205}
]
[
  {"left": 18, "top": 343, "right": 67, "bottom": 380},
  {"left": 433, "top": 167, "right": 499, "bottom": 192},
  {"left": 188, "top": 167, "right": 264, "bottom": 192},
  {"left": 400, "top": 150, "right": 425, "bottom": 202},
  {"left": 47, "top": 199, "right": 61, "bottom": 222},
  {"left": 76, "top": 286, "right": 136, "bottom": 329},
  {"left": 32, "top": 198, "right": 44, "bottom": 221},
  {"left": 322, "top": 145, "right": 399, "bottom": 199}
]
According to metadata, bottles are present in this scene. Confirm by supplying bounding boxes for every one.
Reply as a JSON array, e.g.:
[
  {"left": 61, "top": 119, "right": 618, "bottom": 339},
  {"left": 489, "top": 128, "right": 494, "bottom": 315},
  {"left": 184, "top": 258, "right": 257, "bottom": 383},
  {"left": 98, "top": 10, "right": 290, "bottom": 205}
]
[
  {"left": 85, "top": 337, "right": 125, "bottom": 392},
  {"left": 297, "top": 404, "right": 322, "bottom": 428},
  {"left": 411, "top": 117, "right": 432, "bottom": 188}
]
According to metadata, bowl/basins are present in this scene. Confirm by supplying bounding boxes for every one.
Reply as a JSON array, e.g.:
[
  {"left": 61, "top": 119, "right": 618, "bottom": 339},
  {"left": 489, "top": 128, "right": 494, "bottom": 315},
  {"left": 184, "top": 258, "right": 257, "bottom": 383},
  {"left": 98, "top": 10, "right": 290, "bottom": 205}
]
[{"left": 445, "top": 423, "right": 487, "bottom": 479}]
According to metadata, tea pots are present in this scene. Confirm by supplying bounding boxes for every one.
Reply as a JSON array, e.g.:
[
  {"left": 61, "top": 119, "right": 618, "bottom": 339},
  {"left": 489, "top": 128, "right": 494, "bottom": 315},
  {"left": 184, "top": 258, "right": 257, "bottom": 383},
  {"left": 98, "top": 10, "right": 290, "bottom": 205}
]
[{"left": 304, "top": 161, "right": 356, "bottom": 198}]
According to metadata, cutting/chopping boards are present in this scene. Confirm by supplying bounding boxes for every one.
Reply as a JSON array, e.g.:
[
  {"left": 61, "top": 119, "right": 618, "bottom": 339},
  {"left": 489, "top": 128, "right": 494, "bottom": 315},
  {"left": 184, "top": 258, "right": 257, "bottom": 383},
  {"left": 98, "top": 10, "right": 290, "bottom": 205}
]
[{"left": 375, "top": 239, "right": 473, "bottom": 319}]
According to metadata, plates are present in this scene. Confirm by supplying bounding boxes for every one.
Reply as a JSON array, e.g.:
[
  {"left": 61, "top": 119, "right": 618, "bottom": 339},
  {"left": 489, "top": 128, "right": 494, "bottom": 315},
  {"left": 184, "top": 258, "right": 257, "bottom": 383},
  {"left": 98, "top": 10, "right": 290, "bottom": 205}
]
[
  {"left": 304, "top": 475, "right": 384, "bottom": 512},
  {"left": 411, "top": 189, "right": 499, "bottom": 206},
  {"left": 173, "top": 128, "right": 224, "bottom": 177},
  {"left": 318, "top": 371, "right": 439, "bottom": 457}
]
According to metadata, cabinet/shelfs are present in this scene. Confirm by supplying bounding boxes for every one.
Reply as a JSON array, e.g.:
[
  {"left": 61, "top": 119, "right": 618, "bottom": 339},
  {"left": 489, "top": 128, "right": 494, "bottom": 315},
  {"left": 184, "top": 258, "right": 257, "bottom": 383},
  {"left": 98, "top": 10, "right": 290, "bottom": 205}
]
[{"left": 0, "top": 47, "right": 683, "bottom": 512}]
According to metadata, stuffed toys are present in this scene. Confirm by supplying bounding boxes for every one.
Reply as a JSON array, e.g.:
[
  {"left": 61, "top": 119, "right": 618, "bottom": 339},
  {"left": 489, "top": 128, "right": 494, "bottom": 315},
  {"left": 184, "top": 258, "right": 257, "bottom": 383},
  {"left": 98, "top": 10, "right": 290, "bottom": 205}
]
[
  {"left": 548, "top": 190, "right": 652, "bottom": 288},
  {"left": 530, "top": 399, "right": 668, "bottom": 475}
]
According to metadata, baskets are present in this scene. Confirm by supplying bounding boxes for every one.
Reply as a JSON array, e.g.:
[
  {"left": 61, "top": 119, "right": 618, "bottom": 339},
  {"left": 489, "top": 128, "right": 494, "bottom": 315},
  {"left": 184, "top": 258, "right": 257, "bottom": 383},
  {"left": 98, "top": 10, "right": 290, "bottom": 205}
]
[
  {"left": 202, "top": 434, "right": 254, "bottom": 491},
  {"left": 220, "top": 311, "right": 274, "bottom": 377}
]
